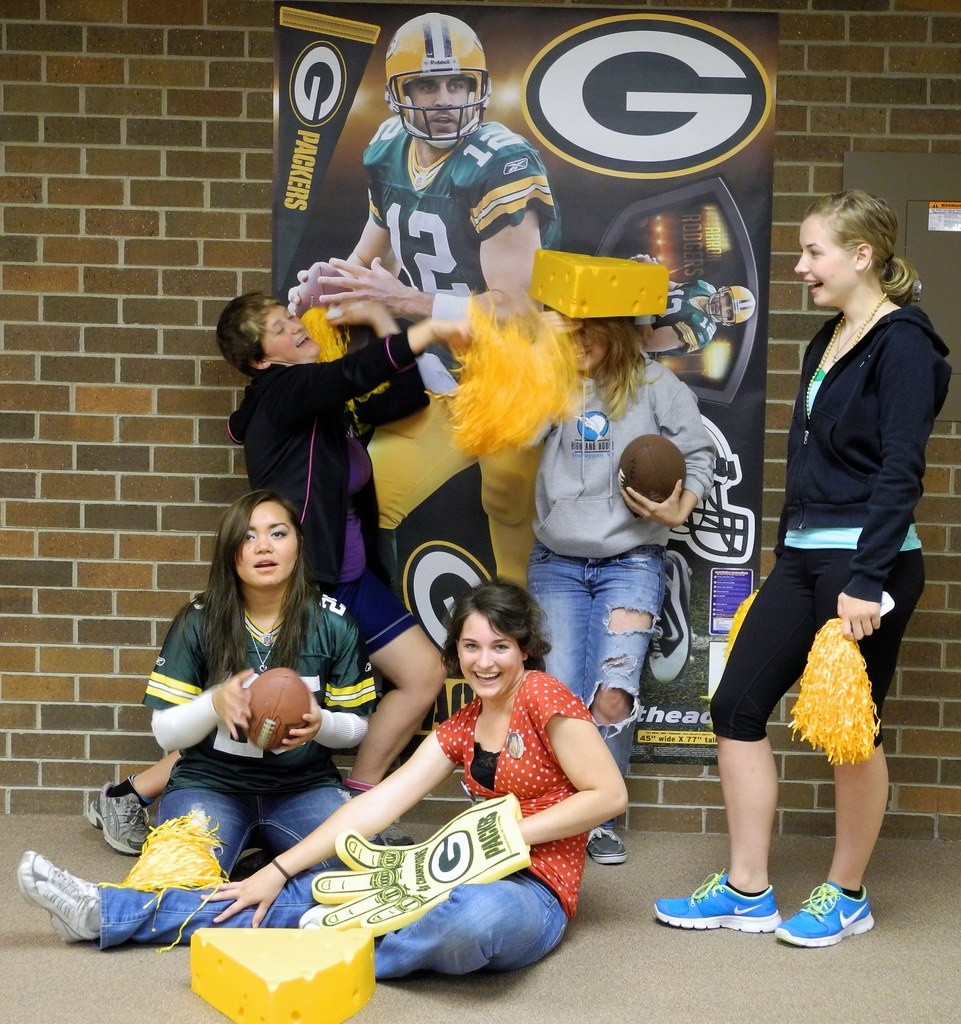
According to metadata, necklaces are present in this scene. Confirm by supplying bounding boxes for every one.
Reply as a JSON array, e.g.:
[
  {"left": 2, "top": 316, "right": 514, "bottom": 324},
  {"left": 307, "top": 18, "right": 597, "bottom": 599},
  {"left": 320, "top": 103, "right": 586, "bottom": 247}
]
[
  {"left": 805, "top": 293, "right": 889, "bottom": 420},
  {"left": 242, "top": 608, "right": 282, "bottom": 673}
]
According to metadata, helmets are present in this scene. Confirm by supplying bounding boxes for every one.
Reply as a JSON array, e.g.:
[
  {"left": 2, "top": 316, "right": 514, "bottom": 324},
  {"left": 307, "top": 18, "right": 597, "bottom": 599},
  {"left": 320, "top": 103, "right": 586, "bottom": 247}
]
[
  {"left": 705, "top": 285, "right": 756, "bottom": 327},
  {"left": 386, "top": 12, "right": 492, "bottom": 150}
]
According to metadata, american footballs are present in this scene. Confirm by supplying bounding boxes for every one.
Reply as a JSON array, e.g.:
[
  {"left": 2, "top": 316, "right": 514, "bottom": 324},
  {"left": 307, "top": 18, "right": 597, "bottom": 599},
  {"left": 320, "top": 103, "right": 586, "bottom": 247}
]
[
  {"left": 244, "top": 668, "right": 311, "bottom": 751},
  {"left": 618, "top": 433, "right": 686, "bottom": 520},
  {"left": 297, "top": 262, "right": 358, "bottom": 349}
]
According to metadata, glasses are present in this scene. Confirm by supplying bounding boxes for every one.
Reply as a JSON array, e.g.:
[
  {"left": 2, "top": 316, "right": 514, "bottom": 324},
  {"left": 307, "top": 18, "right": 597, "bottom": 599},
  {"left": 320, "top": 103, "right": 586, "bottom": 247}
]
[{"left": 571, "top": 329, "right": 594, "bottom": 345}]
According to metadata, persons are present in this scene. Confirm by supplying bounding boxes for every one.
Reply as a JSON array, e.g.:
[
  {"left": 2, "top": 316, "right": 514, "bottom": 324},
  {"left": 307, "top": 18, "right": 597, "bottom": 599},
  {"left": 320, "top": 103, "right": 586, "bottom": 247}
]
[
  {"left": 635, "top": 277, "right": 758, "bottom": 357},
  {"left": 832, "top": 321, "right": 864, "bottom": 363},
  {"left": 15, "top": 575, "right": 629, "bottom": 983},
  {"left": 286, "top": 14, "right": 697, "bottom": 686},
  {"left": 653, "top": 189, "right": 956, "bottom": 950},
  {"left": 141, "top": 490, "right": 389, "bottom": 895},
  {"left": 521, "top": 293, "right": 716, "bottom": 868},
  {"left": 85, "top": 290, "right": 480, "bottom": 857}
]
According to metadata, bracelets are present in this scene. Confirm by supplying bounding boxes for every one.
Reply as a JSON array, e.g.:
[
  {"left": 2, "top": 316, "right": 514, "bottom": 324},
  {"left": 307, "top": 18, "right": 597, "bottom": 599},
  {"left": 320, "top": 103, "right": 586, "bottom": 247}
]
[{"left": 272, "top": 858, "right": 292, "bottom": 880}]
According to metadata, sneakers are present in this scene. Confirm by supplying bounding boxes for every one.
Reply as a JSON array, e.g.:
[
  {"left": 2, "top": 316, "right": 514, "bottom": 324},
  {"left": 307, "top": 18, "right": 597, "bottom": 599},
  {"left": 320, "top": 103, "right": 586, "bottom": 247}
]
[
  {"left": 587, "top": 825, "right": 629, "bottom": 865},
  {"left": 654, "top": 871, "right": 783, "bottom": 934},
  {"left": 642, "top": 551, "right": 690, "bottom": 686},
  {"left": 381, "top": 824, "right": 414, "bottom": 848},
  {"left": 230, "top": 849, "right": 278, "bottom": 880},
  {"left": 299, "top": 904, "right": 340, "bottom": 930},
  {"left": 85, "top": 780, "right": 150, "bottom": 856},
  {"left": 17, "top": 851, "right": 103, "bottom": 945},
  {"left": 774, "top": 879, "right": 879, "bottom": 949}
]
